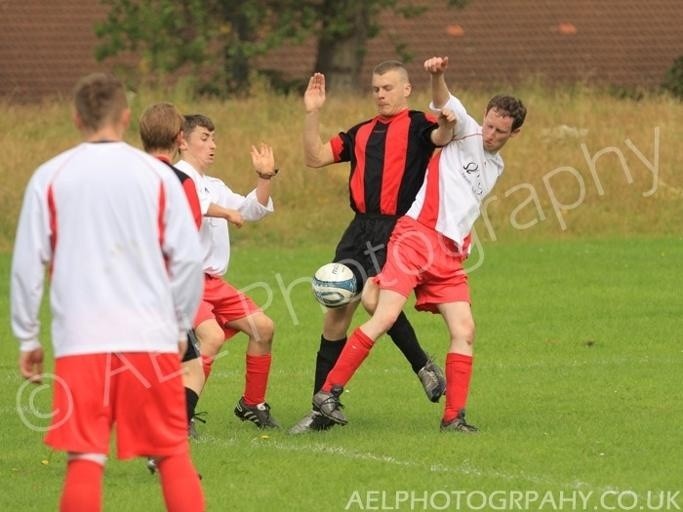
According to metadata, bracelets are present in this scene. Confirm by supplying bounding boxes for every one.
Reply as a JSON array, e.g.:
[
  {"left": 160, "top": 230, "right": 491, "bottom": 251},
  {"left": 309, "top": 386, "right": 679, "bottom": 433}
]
[{"left": 254, "top": 167, "right": 279, "bottom": 180}]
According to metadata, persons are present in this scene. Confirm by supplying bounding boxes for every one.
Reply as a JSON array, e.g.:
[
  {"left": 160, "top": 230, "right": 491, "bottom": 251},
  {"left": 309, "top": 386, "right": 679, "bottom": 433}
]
[
  {"left": 129, "top": 102, "right": 210, "bottom": 483},
  {"left": 287, "top": 60, "right": 459, "bottom": 438},
  {"left": 7, "top": 70, "right": 210, "bottom": 512},
  {"left": 311, "top": 55, "right": 529, "bottom": 433},
  {"left": 168, "top": 112, "right": 282, "bottom": 434}
]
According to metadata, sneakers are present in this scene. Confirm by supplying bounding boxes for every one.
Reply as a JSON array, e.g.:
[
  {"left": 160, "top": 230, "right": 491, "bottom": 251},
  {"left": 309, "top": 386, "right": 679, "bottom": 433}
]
[
  {"left": 311, "top": 385, "right": 348, "bottom": 426},
  {"left": 233, "top": 397, "right": 281, "bottom": 432},
  {"left": 287, "top": 410, "right": 336, "bottom": 436},
  {"left": 439, "top": 413, "right": 481, "bottom": 436},
  {"left": 417, "top": 359, "right": 447, "bottom": 403}
]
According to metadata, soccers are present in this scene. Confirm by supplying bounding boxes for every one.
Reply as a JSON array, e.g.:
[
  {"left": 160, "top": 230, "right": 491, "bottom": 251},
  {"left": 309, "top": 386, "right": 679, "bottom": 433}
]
[{"left": 312, "top": 263, "right": 356, "bottom": 310}]
